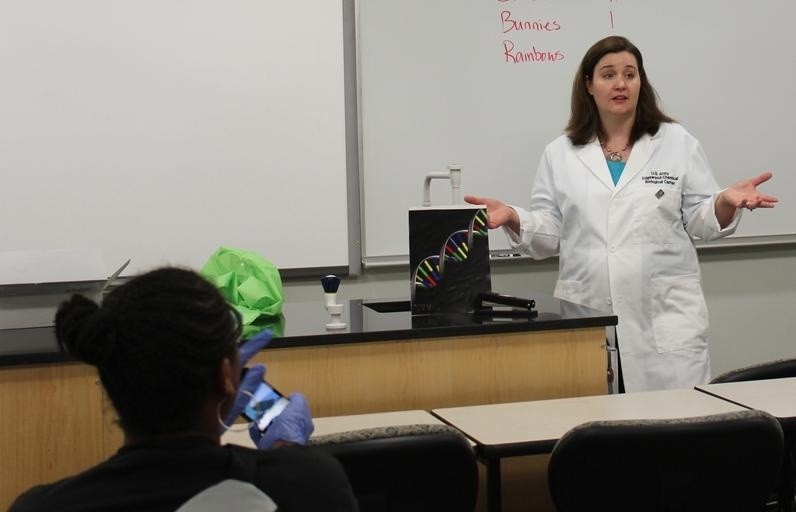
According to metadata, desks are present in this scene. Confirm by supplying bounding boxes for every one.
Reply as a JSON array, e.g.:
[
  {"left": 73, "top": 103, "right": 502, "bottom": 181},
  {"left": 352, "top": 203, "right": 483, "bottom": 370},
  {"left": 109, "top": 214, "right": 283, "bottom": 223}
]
[
  {"left": 694, "top": 376, "right": 796, "bottom": 423},
  {"left": 219, "top": 404, "right": 477, "bottom": 450},
  {"left": 431, "top": 388, "right": 755, "bottom": 511}
]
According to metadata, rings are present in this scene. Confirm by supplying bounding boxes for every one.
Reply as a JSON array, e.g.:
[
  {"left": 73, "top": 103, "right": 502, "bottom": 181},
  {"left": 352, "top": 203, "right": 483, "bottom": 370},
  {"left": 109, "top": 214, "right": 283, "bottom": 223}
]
[{"left": 746, "top": 203, "right": 756, "bottom": 212}]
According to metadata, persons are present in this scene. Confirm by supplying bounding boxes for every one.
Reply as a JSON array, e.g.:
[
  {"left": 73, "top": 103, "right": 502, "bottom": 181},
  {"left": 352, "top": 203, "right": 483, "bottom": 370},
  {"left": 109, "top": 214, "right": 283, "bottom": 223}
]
[
  {"left": 7, "top": 267, "right": 362, "bottom": 512},
  {"left": 463, "top": 35, "right": 780, "bottom": 395}
]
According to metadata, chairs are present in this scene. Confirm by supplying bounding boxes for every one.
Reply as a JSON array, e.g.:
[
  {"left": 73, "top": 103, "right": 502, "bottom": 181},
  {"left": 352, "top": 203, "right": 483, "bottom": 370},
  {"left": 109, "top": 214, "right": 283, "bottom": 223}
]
[
  {"left": 255, "top": 424, "right": 479, "bottom": 511},
  {"left": 546, "top": 409, "right": 785, "bottom": 511},
  {"left": 706, "top": 358, "right": 795, "bottom": 384}
]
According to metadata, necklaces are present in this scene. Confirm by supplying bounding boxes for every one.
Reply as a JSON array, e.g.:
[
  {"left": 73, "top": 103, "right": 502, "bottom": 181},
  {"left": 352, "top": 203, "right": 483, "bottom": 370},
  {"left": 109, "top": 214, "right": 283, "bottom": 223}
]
[{"left": 601, "top": 142, "right": 630, "bottom": 163}]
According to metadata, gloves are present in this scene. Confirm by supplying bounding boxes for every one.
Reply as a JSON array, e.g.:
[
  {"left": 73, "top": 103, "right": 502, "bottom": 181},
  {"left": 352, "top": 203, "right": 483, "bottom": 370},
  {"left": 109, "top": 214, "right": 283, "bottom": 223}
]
[
  {"left": 220, "top": 332, "right": 272, "bottom": 433},
  {"left": 250, "top": 392, "right": 314, "bottom": 449}
]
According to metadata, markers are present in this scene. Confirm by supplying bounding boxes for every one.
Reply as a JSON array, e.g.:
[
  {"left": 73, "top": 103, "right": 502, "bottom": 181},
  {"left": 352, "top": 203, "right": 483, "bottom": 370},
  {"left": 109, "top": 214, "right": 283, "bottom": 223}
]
[{"left": 489, "top": 253, "right": 522, "bottom": 258}]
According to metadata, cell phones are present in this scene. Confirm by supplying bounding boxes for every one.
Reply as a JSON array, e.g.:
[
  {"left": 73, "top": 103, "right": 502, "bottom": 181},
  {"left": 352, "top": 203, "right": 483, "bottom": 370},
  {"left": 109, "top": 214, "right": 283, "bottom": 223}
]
[{"left": 237, "top": 366, "right": 290, "bottom": 436}]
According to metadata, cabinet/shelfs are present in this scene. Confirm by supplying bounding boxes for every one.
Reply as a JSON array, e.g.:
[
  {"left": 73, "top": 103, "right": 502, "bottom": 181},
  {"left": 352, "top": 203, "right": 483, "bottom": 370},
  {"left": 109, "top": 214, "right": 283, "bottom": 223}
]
[{"left": 0, "top": 324, "right": 612, "bottom": 510}]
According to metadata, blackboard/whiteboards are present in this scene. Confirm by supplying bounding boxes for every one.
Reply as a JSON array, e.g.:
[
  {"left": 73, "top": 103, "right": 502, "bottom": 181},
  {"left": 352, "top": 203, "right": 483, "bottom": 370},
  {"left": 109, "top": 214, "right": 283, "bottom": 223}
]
[
  {"left": 353, "top": 0, "right": 796, "bottom": 270},
  {"left": 0, "top": 0, "right": 362, "bottom": 297}
]
